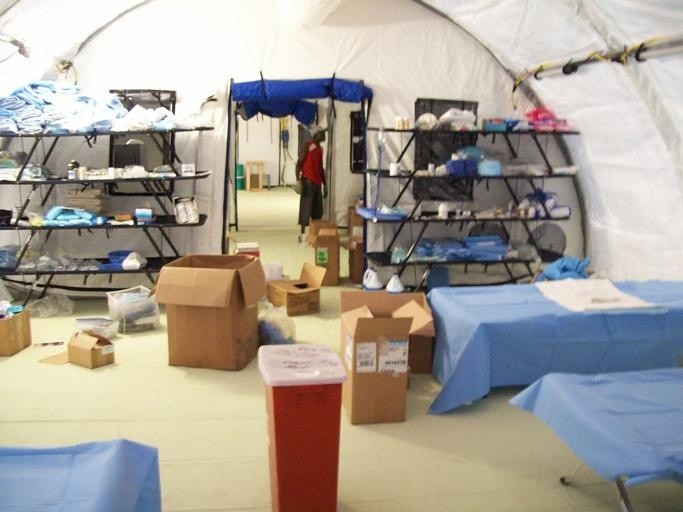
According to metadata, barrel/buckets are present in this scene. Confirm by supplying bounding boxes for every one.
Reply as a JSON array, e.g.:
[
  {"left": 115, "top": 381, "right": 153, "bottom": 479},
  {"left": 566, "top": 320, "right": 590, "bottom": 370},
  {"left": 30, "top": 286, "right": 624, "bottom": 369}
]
[
  {"left": 362, "top": 267, "right": 384, "bottom": 290},
  {"left": 386, "top": 272, "right": 406, "bottom": 293}
]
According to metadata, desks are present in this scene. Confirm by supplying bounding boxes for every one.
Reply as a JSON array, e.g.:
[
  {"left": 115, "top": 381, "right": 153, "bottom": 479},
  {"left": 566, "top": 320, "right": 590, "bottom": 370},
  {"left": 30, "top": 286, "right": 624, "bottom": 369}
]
[
  {"left": 422, "top": 273, "right": 682, "bottom": 423},
  {"left": 0, "top": 435, "right": 164, "bottom": 512},
  {"left": 504, "top": 367, "right": 682, "bottom": 512}
]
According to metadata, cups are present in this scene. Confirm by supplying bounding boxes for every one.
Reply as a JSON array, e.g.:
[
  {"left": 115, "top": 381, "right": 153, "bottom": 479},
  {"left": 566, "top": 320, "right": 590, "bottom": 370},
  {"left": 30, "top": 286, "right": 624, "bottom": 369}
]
[
  {"left": 396, "top": 119, "right": 410, "bottom": 130},
  {"left": 388, "top": 162, "right": 400, "bottom": 176},
  {"left": 79, "top": 167, "right": 86, "bottom": 180},
  {"left": 427, "top": 163, "right": 434, "bottom": 177}
]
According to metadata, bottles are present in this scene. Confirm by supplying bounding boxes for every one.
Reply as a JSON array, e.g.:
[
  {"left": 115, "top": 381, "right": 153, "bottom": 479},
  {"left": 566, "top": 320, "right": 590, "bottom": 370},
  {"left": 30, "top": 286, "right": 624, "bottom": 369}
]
[
  {"left": 438, "top": 204, "right": 447, "bottom": 218},
  {"left": 66, "top": 160, "right": 80, "bottom": 179}
]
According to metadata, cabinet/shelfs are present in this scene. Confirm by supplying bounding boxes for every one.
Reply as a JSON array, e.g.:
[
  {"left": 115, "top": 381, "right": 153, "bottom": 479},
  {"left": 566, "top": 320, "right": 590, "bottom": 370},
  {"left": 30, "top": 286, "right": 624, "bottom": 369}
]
[
  {"left": 0, "top": 123, "right": 218, "bottom": 310},
  {"left": 351, "top": 121, "right": 586, "bottom": 295}
]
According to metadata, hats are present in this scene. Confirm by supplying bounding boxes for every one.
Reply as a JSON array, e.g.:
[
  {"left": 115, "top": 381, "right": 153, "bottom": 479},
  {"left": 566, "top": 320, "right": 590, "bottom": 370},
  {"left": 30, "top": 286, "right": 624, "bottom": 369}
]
[{"left": 310, "top": 124, "right": 329, "bottom": 138}]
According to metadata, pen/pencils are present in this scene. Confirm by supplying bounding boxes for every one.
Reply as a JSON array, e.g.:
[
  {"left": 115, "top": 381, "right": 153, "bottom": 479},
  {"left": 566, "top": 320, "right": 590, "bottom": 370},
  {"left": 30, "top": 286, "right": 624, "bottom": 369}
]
[
  {"left": 41, "top": 342, "right": 64, "bottom": 346},
  {"left": 591, "top": 299, "right": 619, "bottom": 302}
]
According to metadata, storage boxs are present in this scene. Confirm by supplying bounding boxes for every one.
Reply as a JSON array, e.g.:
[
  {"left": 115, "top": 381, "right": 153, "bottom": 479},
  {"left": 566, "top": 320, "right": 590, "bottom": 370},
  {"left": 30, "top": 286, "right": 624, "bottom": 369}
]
[
  {"left": 104, "top": 283, "right": 163, "bottom": 335},
  {"left": 0, "top": 303, "right": 36, "bottom": 360},
  {"left": 62, "top": 328, "right": 117, "bottom": 368},
  {"left": 339, "top": 298, "right": 433, "bottom": 428},
  {"left": 345, "top": 204, "right": 365, "bottom": 285},
  {"left": 151, "top": 252, "right": 271, "bottom": 373},
  {"left": 264, "top": 260, "right": 330, "bottom": 318},
  {"left": 307, "top": 215, "right": 342, "bottom": 286},
  {"left": 336, "top": 289, "right": 437, "bottom": 375}
]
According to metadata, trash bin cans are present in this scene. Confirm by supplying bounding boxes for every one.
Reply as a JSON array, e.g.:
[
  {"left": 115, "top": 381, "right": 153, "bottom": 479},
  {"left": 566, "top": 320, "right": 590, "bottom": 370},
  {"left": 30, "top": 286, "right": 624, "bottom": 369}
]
[{"left": 236, "top": 164, "right": 244, "bottom": 189}]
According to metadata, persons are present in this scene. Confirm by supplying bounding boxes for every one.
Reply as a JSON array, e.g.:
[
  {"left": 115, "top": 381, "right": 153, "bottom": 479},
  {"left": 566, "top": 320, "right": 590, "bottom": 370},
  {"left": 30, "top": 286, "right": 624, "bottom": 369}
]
[{"left": 295, "top": 125, "right": 329, "bottom": 244}]
